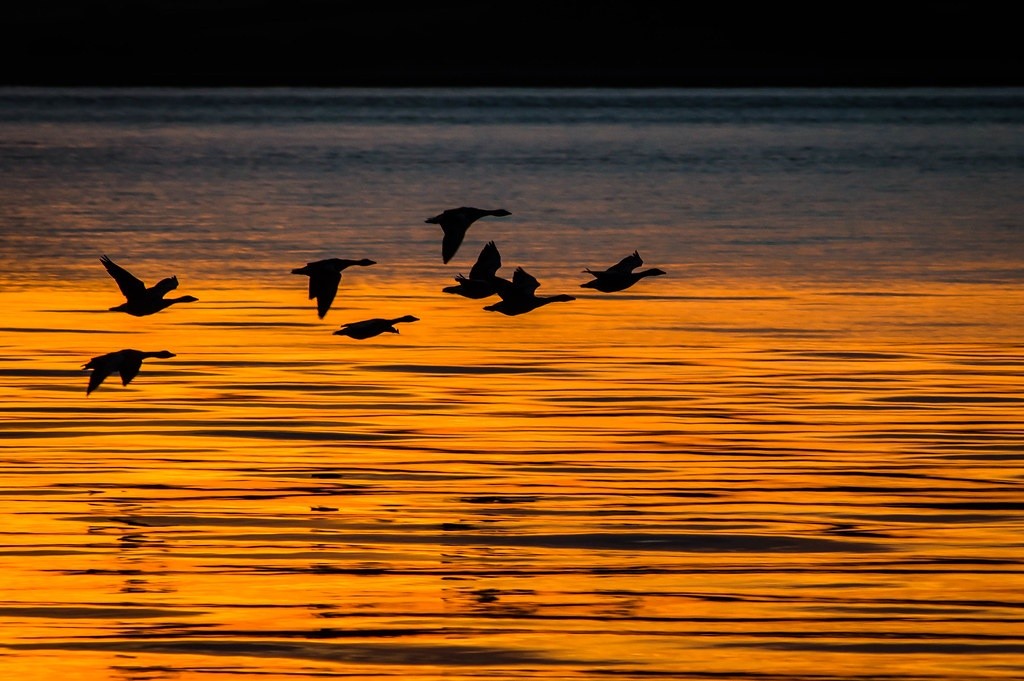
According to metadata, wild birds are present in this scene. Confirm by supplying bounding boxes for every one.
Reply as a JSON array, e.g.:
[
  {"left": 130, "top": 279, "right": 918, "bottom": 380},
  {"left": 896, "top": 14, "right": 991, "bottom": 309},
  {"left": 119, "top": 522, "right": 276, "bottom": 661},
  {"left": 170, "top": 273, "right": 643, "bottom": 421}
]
[
  {"left": 577, "top": 250, "right": 667, "bottom": 296},
  {"left": 99, "top": 254, "right": 199, "bottom": 317},
  {"left": 441, "top": 241, "right": 576, "bottom": 316},
  {"left": 424, "top": 207, "right": 513, "bottom": 264},
  {"left": 81, "top": 347, "right": 176, "bottom": 396},
  {"left": 331, "top": 314, "right": 421, "bottom": 340},
  {"left": 291, "top": 257, "right": 378, "bottom": 320}
]
